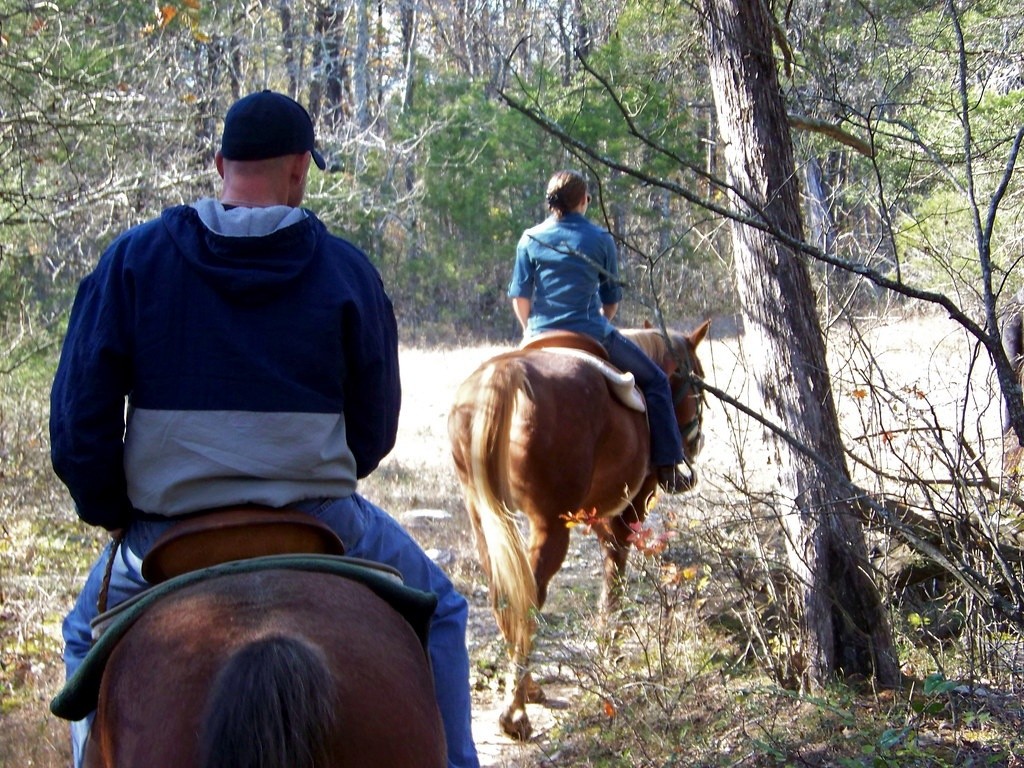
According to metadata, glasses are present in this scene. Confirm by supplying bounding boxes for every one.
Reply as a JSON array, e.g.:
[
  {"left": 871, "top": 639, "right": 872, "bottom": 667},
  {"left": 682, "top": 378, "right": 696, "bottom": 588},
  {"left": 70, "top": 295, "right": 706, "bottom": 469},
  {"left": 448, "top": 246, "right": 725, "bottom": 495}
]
[{"left": 587, "top": 196, "right": 592, "bottom": 202}]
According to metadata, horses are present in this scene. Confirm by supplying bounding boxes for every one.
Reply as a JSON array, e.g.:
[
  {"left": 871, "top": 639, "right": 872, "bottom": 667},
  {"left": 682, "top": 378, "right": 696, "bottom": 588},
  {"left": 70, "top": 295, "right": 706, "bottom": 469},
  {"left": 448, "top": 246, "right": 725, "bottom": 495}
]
[
  {"left": 75, "top": 565, "right": 449, "bottom": 768},
  {"left": 446, "top": 317, "right": 710, "bottom": 738}
]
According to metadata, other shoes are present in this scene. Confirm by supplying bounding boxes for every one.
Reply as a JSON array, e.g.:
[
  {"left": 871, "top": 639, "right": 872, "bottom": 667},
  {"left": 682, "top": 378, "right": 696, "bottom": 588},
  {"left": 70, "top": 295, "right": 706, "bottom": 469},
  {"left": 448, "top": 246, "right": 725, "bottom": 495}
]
[{"left": 658, "top": 457, "right": 696, "bottom": 494}]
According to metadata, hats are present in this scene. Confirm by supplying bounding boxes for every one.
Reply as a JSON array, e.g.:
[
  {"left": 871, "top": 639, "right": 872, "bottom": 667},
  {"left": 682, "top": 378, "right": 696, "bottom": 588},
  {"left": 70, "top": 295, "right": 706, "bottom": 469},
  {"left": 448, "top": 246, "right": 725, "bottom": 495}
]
[{"left": 221, "top": 89, "right": 327, "bottom": 171}]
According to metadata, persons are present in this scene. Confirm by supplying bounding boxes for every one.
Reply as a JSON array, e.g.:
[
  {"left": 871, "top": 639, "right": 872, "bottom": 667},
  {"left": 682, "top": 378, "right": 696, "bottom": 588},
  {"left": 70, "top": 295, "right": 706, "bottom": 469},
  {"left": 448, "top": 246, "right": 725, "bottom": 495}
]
[
  {"left": 506, "top": 171, "right": 689, "bottom": 495},
  {"left": 48, "top": 90, "right": 481, "bottom": 768}
]
